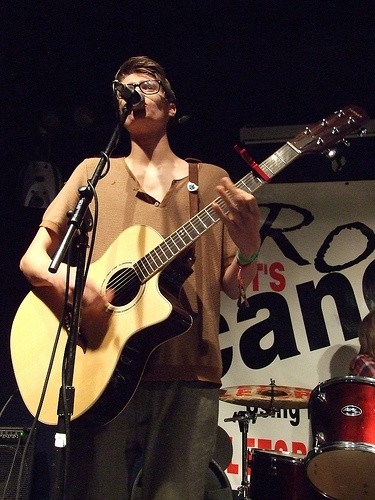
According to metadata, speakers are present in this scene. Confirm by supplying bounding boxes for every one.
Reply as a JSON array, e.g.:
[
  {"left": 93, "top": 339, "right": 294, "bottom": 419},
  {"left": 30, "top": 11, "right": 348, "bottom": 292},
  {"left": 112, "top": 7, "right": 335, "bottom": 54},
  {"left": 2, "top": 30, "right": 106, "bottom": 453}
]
[{"left": 0, "top": 426, "right": 37, "bottom": 500}]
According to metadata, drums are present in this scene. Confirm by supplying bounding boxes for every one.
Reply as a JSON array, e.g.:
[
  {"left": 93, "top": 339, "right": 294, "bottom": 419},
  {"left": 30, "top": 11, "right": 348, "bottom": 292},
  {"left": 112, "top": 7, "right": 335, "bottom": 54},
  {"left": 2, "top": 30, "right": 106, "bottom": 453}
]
[
  {"left": 245, "top": 446, "right": 313, "bottom": 500},
  {"left": 304, "top": 376, "right": 375, "bottom": 500},
  {"left": 131, "top": 456, "right": 231, "bottom": 500}
]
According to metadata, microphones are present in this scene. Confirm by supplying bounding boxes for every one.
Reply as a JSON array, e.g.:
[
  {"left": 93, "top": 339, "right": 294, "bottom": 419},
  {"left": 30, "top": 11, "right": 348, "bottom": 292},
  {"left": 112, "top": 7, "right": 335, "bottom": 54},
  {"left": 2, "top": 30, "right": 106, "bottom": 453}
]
[{"left": 113, "top": 80, "right": 146, "bottom": 112}]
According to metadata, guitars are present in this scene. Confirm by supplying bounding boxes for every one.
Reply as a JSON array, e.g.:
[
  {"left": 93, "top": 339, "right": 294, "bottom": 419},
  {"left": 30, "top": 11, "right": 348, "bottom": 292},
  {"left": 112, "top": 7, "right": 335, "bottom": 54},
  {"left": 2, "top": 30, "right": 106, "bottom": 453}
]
[{"left": 10, "top": 105, "right": 370, "bottom": 432}]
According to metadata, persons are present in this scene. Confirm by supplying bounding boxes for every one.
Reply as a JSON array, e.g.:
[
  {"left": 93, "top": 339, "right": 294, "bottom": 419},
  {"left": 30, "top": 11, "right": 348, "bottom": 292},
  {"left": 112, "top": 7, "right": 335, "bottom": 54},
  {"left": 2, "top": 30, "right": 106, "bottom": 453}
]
[
  {"left": 349, "top": 309, "right": 375, "bottom": 380},
  {"left": 19, "top": 56, "right": 263, "bottom": 500}
]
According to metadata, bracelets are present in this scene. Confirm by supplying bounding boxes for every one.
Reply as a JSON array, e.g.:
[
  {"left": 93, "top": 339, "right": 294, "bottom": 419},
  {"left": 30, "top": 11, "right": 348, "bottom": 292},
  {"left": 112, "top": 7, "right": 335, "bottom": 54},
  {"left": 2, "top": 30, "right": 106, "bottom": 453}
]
[{"left": 235, "top": 246, "right": 262, "bottom": 308}]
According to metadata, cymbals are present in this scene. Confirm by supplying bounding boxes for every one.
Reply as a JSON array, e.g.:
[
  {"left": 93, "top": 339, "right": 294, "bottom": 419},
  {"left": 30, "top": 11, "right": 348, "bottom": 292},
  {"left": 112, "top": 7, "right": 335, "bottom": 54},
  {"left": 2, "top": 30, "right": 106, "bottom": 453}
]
[
  {"left": 212, "top": 425, "right": 232, "bottom": 472},
  {"left": 218, "top": 384, "right": 315, "bottom": 410}
]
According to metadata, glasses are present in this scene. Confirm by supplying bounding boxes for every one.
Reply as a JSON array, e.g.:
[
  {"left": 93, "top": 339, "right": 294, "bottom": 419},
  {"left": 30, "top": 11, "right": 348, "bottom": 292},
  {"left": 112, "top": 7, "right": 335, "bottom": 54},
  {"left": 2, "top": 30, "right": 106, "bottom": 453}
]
[{"left": 115, "top": 79, "right": 165, "bottom": 99}]
